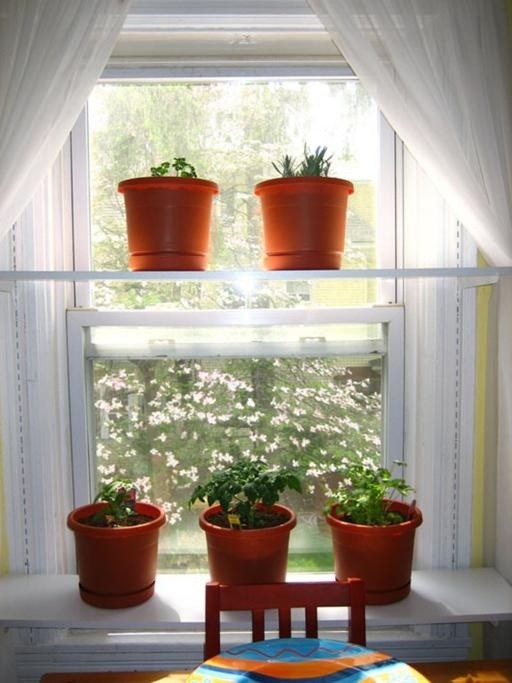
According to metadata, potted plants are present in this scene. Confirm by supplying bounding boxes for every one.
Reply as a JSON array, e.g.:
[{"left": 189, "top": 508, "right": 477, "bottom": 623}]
[
  {"left": 319, "top": 446, "right": 427, "bottom": 606},
  {"left": 182, "top": 455, "right": 309, "bottom": 588},
  {"left": 115, "top": 153, "right": 224, "bottom": 272},
  {"left": 67, "top": 473, "right": 170, "bottom": 612},
  {"left": 250, "top": 140, "right": 356, "bottom": 270}
]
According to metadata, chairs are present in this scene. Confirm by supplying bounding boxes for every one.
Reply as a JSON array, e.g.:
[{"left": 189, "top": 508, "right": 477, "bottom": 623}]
[{"left": 202, "top": 574, "right": 368, "bottom": 662}]
